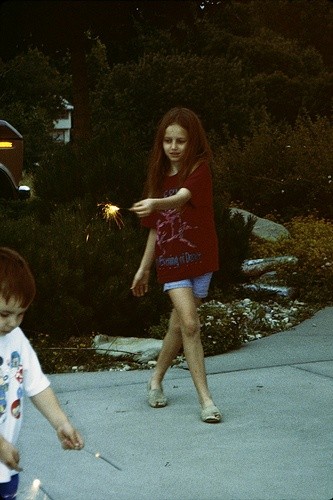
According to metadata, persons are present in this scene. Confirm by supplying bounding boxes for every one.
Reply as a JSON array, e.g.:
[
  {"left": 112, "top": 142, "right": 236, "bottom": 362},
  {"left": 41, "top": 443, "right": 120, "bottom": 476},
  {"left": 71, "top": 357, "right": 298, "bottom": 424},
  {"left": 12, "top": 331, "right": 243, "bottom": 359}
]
[
  {"left": 129, "top": 108, "right": 222, "bottom": 423},
  {"left": 0, "top": 248, "right": 86, "bottom": 500}
]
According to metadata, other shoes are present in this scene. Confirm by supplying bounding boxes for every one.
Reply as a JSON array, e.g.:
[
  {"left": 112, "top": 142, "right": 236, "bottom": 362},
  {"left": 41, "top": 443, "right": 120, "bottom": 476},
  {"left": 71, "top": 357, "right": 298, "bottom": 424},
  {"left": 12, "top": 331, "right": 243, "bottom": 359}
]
[
  {"left": 147, "top": 378, "right": 167, "bottom": 407},
  {"left": 201, "top": 405, "right": 222, "bottom": 423}
]
